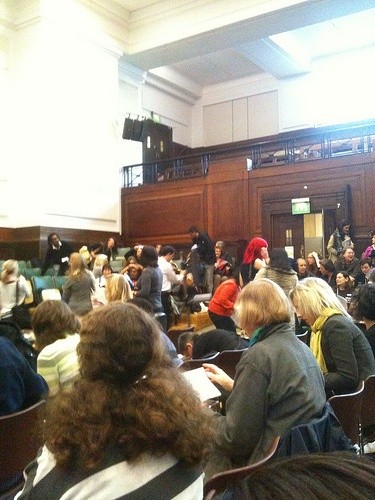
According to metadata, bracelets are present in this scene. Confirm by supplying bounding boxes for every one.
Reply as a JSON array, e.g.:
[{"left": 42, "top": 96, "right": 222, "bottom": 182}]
[{"left": 127, "top": 265, "right": 131, "bottom": 270}]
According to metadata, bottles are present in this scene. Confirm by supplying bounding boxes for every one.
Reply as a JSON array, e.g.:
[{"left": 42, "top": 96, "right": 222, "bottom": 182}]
[
  {"left": 355, "top": 282, "right": 362, "bottom": 293},
  {"left": 345, "top": 293, "right": 352, "bottom": 308}
]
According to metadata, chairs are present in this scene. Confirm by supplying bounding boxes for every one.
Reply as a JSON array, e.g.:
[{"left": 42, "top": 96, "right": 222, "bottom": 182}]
[{"left": 0, "top": 247, "right": 375, "bottom": 500}]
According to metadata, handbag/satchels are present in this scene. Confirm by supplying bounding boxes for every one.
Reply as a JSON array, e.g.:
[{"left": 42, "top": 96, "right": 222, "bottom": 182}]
[{"left": 12, "top": 280, "right": 38, "bottom": 329}]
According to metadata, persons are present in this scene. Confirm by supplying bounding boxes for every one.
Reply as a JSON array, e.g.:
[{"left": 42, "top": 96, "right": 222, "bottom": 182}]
[{"left": 0, "top": 219, "right": 375, "bottom": 500}]
